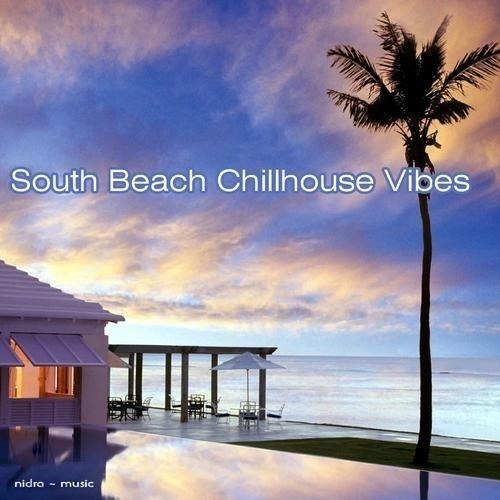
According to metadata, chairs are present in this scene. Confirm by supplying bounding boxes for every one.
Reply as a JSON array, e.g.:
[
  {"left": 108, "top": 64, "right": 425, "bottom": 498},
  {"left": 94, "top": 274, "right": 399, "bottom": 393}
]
[
  {"left": 108, "top": 395, "right": 152, "bottom": 421},
  {"left": 168, "top": 394, "right": 285, "bottom": 431}
]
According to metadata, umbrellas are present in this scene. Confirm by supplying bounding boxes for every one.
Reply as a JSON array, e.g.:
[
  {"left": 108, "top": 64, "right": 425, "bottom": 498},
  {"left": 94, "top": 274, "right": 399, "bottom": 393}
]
[{"left": 208, "top": 352, "right": 287, "bottom": 430}]
